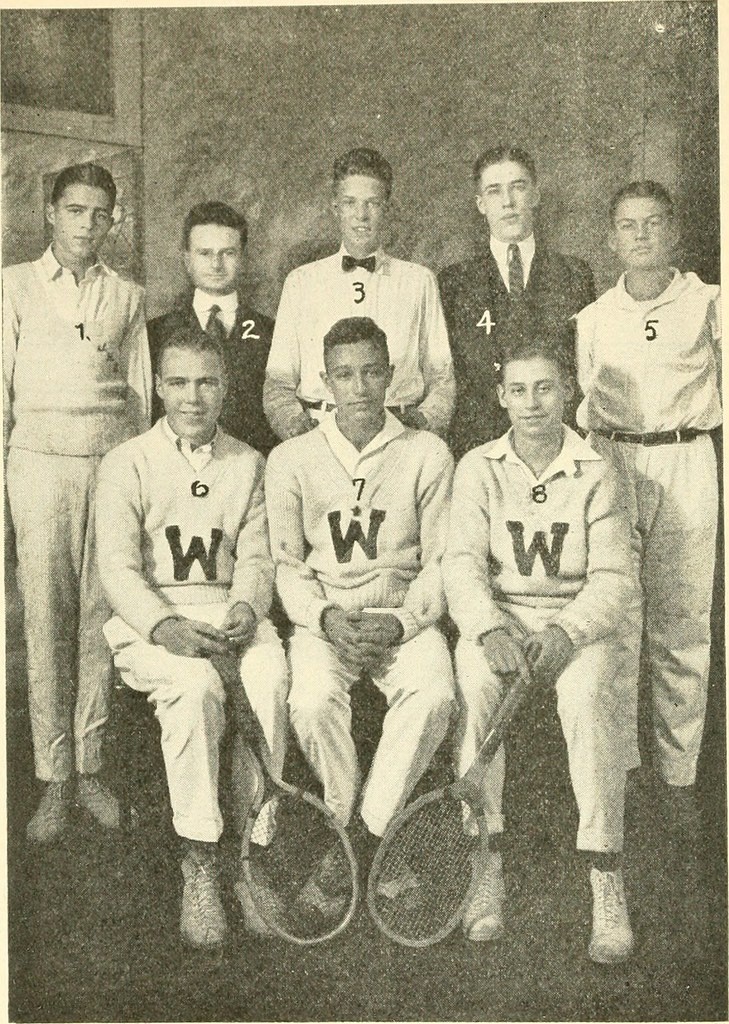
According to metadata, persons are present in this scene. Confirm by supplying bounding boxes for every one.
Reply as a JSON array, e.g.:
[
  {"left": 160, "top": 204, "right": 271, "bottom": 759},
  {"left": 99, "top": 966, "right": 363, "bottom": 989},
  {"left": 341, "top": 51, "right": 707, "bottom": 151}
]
[
  {"left": 429, "top": 146, "right": 597, "bottom": 466},
  {"left": 261, "top": 315, "right": 458, "bottom": 924},
  {"left": 260, "top": 148, "right": 461, "bottom": 441},
  {"left": 572, "top": 179, "right": 722, "bottom": 824},
  {"left": 3, "top": 161, "right": 156, "bottom": 844},
  {"left": 92, "top": 329, "right": 298, "bottom": 948},
  {"left": 443, "top": 336, "right": 642, "bottom": 971},
  {"left": 142, "top": 201, "right": 283, "bottom": 459}
]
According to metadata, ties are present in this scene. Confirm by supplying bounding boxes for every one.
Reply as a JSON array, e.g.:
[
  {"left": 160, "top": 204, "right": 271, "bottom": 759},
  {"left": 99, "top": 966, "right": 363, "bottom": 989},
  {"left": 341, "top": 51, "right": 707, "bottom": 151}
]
[
  {"left": 507, "top": 243, "right": 525, "bottom": 334},
  {"left": 204, "top": 304, "right": 228, "bottom": 346}
]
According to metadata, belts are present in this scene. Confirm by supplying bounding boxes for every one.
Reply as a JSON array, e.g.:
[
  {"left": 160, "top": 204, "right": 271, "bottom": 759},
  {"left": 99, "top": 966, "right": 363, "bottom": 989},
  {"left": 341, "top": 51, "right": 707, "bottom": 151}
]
[
  {"left": 303, "top": 399, "right": 422, "bottom": 416},
  {"left": 592, "top": 427, "right": 707, "bottom": 447}
]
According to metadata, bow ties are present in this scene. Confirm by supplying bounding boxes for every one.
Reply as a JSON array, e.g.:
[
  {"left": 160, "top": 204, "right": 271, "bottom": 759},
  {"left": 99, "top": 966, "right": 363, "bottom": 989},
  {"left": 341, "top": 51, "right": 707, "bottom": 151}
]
[{"left": 341, "top": 255, "right": 376, "bottom": 273}]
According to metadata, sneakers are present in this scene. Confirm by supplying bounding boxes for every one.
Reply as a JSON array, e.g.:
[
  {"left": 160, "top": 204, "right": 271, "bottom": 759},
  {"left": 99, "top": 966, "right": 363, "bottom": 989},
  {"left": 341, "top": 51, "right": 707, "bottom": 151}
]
[
  {"left": 180, "top": 855, "right": 230, "bottom": 952},
  {"left": 461, "top": 850, "right": 507, "bottom": 942},
  {"left": 291, "top": 823, "right": 424, "bottom": 934},
  {"left": 588, "top": 867, "right": 635, "bottom": 965},
  {"left": 74, "top": 775, "right": 139, "bottom": 832},
  {"left": 26, "top": 779, "right": 74, "bottom": 845},
  {"left": 234, "top": 875, "right": 287, "bottom": 938}
]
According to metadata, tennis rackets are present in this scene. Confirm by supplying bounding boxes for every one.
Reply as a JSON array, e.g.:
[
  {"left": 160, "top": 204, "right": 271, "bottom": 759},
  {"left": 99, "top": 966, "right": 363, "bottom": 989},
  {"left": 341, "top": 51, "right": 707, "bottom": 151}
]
[
  {"left": 209, "top": 648, "right": 357, "bottom": 948},
  {"left": 367, "top": 636, "right": 547, "bottom": 946}
]
